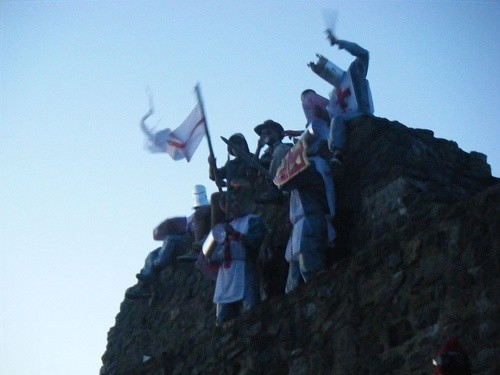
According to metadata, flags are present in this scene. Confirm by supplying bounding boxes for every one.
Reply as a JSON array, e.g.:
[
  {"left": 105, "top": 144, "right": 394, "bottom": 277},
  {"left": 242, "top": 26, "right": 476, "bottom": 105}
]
[{"left": 166, "top": 106, "right": 209, "bottom": 160}]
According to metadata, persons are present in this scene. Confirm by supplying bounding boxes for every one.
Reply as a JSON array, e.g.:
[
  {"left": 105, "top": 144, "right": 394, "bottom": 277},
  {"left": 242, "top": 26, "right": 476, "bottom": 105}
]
[
  {"left": 209, "top": 193, "right": 270, "bottom": 329},
  {"left": 126, "top": 182, "right": 208, "bottom": 301},
  {"left": 304, "top": 26, "right": 373, "bottom": 171},
  {"left": 255, "top": 122, "right": 297, "bottom": 162},
  {"left": 268, "top": 141, "right": 338, "bottom": 293},
  {"left": 179, "top": 133, "right": 257, "bottom": 262},
  {"left": 292, "top": 89, "right": 319, "bottom": 143}
]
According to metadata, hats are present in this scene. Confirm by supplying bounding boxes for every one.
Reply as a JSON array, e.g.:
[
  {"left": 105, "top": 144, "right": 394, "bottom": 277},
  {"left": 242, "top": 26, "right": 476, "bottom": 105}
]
[
  {"left": 254, "top": 120, "right": 284, "bottom": 139},
  {"left": 226, "top": 133, "right": 246, "bottom": 154}
]
